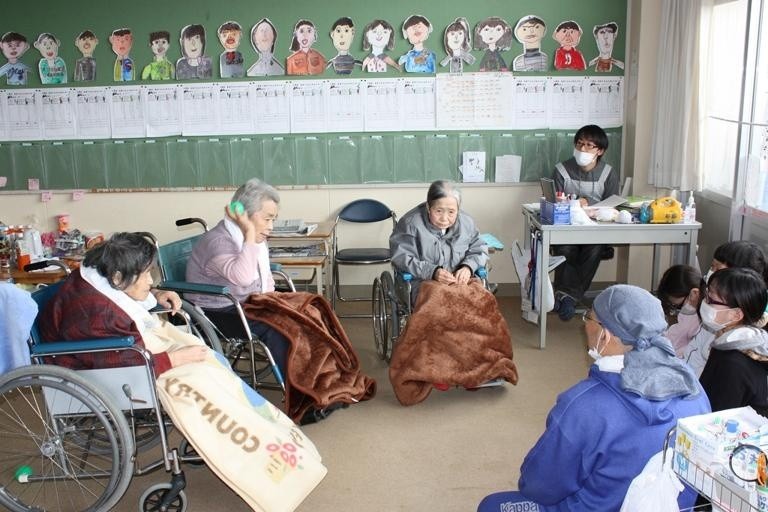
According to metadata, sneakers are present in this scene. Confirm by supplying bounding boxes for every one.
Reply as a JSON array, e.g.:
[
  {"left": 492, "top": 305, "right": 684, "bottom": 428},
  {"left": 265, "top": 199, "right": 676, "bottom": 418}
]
[{"left": 560, "top": 297, "right": 577, "bottom": 320}]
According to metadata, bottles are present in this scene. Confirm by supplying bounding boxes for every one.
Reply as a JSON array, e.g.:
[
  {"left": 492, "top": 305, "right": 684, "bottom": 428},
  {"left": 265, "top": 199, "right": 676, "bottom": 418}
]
[
  {"left": 684, "top": 191, "right": 696, "bottom": 224},
  {"left": 0, "top": 224, "right": 33, "bottom": 271}
]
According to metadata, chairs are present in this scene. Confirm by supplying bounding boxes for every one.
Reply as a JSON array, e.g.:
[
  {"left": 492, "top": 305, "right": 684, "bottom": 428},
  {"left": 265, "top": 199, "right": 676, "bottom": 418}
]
[{"left": 332, "top": 199, "right": 400, "bottom": 319}]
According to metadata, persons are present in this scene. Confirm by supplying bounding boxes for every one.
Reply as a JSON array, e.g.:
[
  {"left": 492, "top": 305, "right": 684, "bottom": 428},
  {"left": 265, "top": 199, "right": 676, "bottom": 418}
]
[
  {"left": 698, "top": 267, "right": 767, "bottom": 418},
  {"left": 658, "top": 266, "right": 705, "bottom": 358},
  {"left": 439, "top": 18, "right": 476, "bottom": 73},
  {"left": 246, "top": 18, "right": 285, "bottom": 76},
  {"left": 37, "top": 233, "right": 210, "bottom": 380},
  {"left": 397, "top": 16, "right": 436, "bottom": 73},
  {"left": 389, "top": 180, "right": 490, "bottom": 393},
  {"left": 109, "top": 27, "right": 134, "bottom": 81},
  {"left": 285, "top": 19, "right": 325, "bottom": 75},
  {"left": 327, "top": 18, "right": 363, "bottom": 74},
  {"left": 474, "top": 17, "right": 512, "bottom": 71},
  {"left": 216, "top": 21, "right": 245, "bottom": 78},
  {"left": 552, "top": 20, "right": 587, "bottom": 70},
  {"left": 184, "top": 178, "right": 293, "bottom": 381},
  {"left": 512, "top": 15, "right": 547, "bottom": 72},
  {"left": 701, "top": 240, "right": 768, "bottom": 278},
  {"left": 0, "top": 31, "right": 31, "bottom": 86},
  {"left": 75, "top": 31, "right": 99, "bottom": 82},
  {"left": 141, "top": 31, "right": 176, "bottom": 81},
  {"left": 176, "top": 25, "right": 211, "bottom": 79},
  {"left": 551, "top": 124, "right": 619, "bottom": 321},
  {"left": 33, "top": 33, "right": 68, "bottom": 85},
  {"left": 361, "top": 21, "right": 397, "bottom": 71},
  {"left": 589, "top": 22, "right": 624, "bottom": 73},
  {"left": 477, "top": 285, "right": 713, "bottom": 512}
]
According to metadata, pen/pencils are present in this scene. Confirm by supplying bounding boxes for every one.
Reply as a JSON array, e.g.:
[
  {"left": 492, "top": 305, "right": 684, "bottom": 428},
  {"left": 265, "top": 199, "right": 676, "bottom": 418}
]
[
  {"left": 556, "top": 191, "right": 569, "bottom": 203},
  {"left": 567, "top": 194, "right": 579, "bottom": 201}
]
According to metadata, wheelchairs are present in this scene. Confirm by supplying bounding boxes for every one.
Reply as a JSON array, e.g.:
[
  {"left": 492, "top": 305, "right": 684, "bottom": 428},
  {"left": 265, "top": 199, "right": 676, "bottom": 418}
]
[
  {"left": 372, "top": 211, "right": 505, "bottom": 388},
  {"left": 133, "top": 218, "right": 343, "bottom": 425},
  {"left": 0, "top": 260, "right": 225, "bottom": 512}
]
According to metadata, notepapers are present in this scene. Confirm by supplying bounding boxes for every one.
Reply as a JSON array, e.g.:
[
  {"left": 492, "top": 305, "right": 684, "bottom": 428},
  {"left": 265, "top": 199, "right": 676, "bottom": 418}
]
[
  {"left": 73, "top": 191, "right": 83, "bottom": 201},
  {"left": 28, "top": 179, "right": 39, "bottom": 190},
  {"left": 0, "top": 177, "right": 7, "bottom": 187},
  {"left": 42, "top": 192, "right": 53, "bottom": 202}
]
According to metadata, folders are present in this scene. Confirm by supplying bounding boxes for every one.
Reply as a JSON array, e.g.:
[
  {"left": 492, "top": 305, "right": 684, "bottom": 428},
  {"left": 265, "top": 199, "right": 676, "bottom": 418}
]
[{"left": 540, "top": 177, "right": 556, "bottom": 202}]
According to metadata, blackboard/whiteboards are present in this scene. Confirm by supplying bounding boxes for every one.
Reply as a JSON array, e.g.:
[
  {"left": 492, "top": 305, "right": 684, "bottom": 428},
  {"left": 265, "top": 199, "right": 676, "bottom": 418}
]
[{"left": 0, "top": 0, "right": 629, "bottom": 195}]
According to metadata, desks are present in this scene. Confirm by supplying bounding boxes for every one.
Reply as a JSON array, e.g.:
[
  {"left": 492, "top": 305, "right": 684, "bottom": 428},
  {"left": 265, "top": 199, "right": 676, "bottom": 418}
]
[
  {"left": 520, "top": 202, "right": 703, "bottom": 350},
  {"left": 0, "top": 259, "right": 82, "bottom": 285},
  {"left": 266, "top": 222, "right": 336, "bottom": 302},
  {"left": 268, "top": 256, "right": 328, "bottom": 296}
]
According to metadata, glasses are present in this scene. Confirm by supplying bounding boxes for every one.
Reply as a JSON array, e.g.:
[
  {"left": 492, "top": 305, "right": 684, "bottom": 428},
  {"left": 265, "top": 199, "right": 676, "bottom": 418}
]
[
  {"left": 703, "top": 286, "right": 737, "bottom": 309},
  {"left": 666, "top": 287, "right": 692, "bottom": 311},
  {"left": 709, "top": 263, "right": 724, "bottom": 271},
  {"left": 574, "top": 140, "right": 602, "bottom": 150},
  {"left": 583, "top": 308, "right": 606, "bottom": 329}
]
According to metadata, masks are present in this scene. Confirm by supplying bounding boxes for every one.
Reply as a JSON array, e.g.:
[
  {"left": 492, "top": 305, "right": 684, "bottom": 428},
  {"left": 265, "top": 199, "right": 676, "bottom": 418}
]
[
  {"left": 587, "top": 329, "right": 611, "bottom": 361},
  {"left": 572, "top": 148, "right": 599, "bottom": 167},
  {"left": 699, "top": 298, "right": 738, "bottom": 332},
  {"left": 679, "top": 293, "right": 697, "bottom": 315},
  {"left": 705, "top": 268, "right": 714, "bottom": 285}
]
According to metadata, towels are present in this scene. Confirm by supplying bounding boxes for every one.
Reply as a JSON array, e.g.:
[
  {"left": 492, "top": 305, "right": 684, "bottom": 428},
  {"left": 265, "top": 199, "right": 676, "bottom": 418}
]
[
  {"left": 222, "top": 209, "right": 277, "bottom": 295},
  {"left": 78, "top": 255, "right": 177, "bottom": 355}
]
